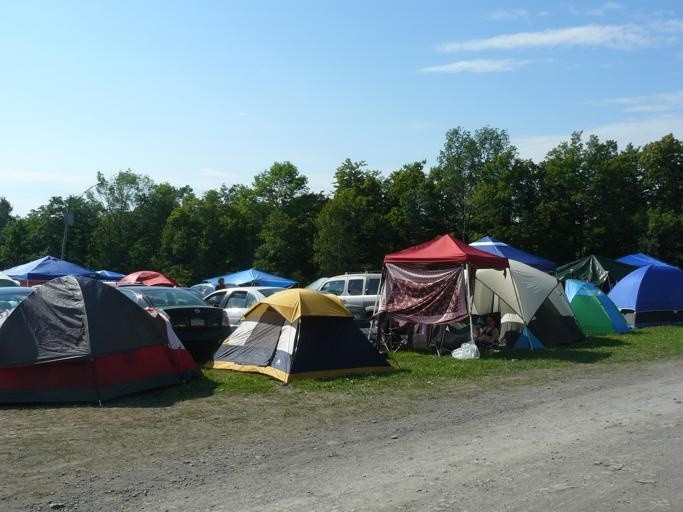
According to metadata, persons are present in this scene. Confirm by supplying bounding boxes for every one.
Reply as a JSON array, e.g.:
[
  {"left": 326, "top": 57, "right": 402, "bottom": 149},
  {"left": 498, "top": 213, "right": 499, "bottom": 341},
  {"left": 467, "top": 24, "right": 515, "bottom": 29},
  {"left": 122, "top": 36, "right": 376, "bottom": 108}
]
[
  {"left": 474, "top": 314, "right": 499, "bottom": 344},
  {"left": 215, "top": 278, "right": 225, "bottom": 307}
]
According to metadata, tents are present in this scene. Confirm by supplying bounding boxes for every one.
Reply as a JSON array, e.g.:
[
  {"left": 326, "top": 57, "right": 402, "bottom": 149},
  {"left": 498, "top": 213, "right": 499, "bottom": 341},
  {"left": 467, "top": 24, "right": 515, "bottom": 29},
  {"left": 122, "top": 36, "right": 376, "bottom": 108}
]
[
  {"left": 565, "top": 278, "right": 629, "bottom": 335},
  {"left": 608, "top": 264, "right": 683, "bottom": 329},
  {"left": 470, "top": 235, "right": 586, "bottom": 351},
  {"left": 198, "top": 288, "right": 397, "bottom": 385},
  {"left": 2, "top": 274, "right": 204, "bottom": 406},
  {"left": 1, "top": 253, "right": 179, "bottom": 287}
]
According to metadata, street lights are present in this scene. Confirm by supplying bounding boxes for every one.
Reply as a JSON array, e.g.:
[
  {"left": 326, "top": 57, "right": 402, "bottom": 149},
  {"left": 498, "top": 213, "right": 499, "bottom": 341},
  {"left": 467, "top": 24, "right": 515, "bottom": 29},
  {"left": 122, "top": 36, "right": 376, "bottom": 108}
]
[{"left": 60, "top": 181, "right": 105, "bottom": 261}]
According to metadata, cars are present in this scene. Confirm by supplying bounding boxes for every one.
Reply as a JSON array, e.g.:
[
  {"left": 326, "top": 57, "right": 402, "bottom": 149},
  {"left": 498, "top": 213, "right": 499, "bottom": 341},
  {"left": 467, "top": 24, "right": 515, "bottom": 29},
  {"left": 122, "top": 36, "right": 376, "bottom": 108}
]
[
  {"left": 0, "top": 286, "right": 34, "bottom": 316},
  {"left": 202, "top": 287, "right": 288, "bottom": 332},
  {"left": 189, "top": 283, "right": 241, "bottom": 301},
  {"left": 116, "top": 286, "right": 230, "bottom": 354}
]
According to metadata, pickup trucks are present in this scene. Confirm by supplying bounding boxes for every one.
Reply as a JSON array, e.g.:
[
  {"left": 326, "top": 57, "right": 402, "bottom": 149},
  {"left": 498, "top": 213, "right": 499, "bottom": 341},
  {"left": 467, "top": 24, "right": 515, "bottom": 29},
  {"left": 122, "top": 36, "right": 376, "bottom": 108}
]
[{"left": 317, "top": 273, "right": 381, "bottom": 319}]
